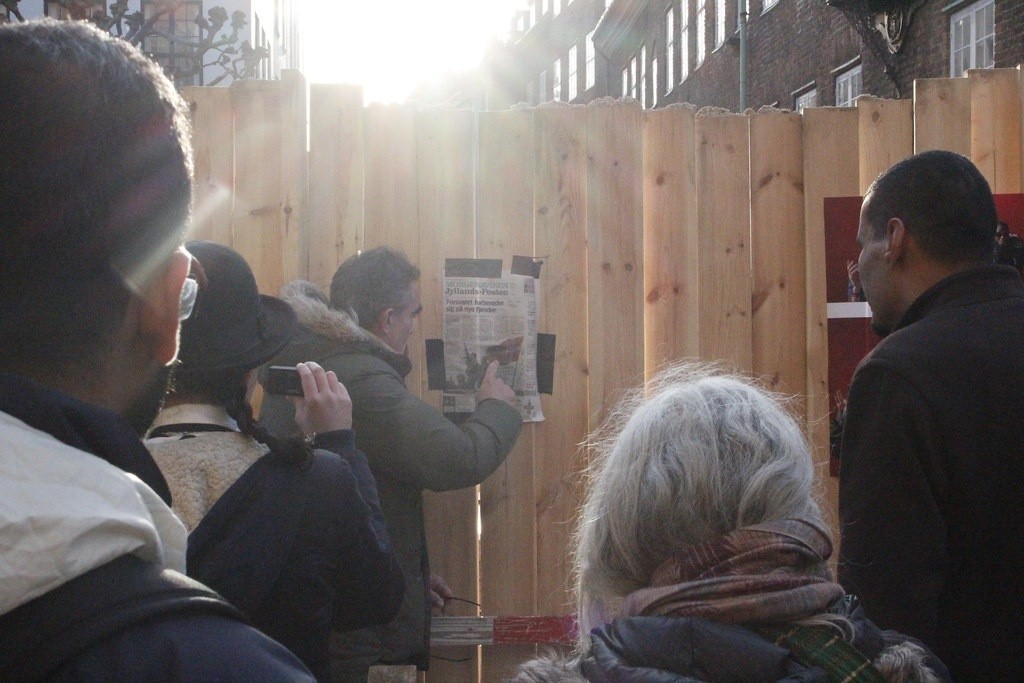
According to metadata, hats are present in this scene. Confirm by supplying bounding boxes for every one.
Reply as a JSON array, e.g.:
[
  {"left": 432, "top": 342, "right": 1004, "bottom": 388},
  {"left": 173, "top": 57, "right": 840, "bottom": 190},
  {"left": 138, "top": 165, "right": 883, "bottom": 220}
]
[{"left": 170, "top": 240, "right": 297, "bottom": 378}]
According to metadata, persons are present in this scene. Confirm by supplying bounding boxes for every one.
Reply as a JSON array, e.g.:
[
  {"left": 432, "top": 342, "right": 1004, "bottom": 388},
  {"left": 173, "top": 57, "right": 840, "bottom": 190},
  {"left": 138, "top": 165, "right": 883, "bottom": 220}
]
[
  {"left": 846, "top": 259, "right": 866, "bottom": 302},
  {"left": 139, "top": 240, "right": 407, "bottom": 683},
  {"left": 836, "top": 149, "right": 1024, "bottom": 683},
  {"left": 259, "top": 245, "right": 523, "bottom": 683},
  {"left": 991, "top": 221, "right": 1024, "bottom": 267},
  {"left": 0, "top": 19, "right": 313, "bottom": 683},
  {"left": 516, "top": 361, "right": 947, "bottom": 683}
]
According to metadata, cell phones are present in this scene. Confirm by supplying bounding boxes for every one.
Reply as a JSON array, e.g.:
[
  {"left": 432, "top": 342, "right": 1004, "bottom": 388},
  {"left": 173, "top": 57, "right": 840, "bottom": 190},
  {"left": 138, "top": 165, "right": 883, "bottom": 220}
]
[{"left": 268, "top": 366, "right": 304, "bottom": 397}]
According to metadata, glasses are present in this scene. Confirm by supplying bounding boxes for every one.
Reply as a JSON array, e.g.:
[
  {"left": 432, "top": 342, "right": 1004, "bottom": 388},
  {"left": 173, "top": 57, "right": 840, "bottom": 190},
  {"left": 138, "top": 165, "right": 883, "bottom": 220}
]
[{"left": 173, "top": 244, "right": 210, "bottom": 321}]
